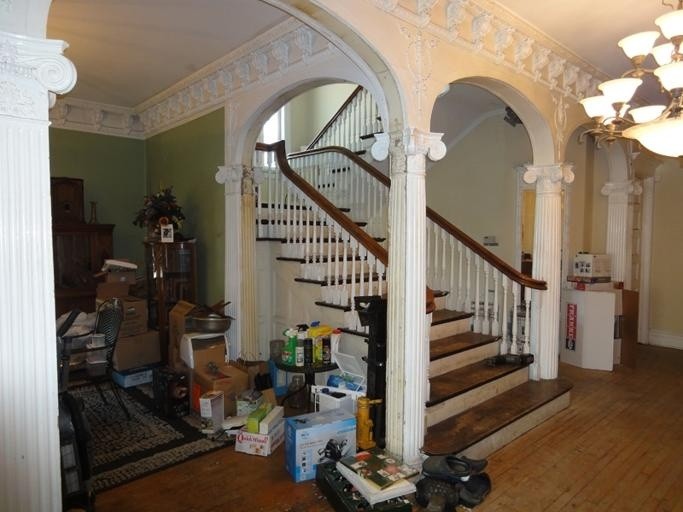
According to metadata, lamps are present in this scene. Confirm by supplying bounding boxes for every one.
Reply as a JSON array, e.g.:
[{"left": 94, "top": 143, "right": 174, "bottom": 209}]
[{"left": 575, "top": 1, "right": 683, "bottom": 161}]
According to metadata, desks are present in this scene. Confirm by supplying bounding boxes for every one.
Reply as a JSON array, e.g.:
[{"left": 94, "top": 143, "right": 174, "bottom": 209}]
[{"left": 275, "top": 354, "right": 339, "bottom": 413}]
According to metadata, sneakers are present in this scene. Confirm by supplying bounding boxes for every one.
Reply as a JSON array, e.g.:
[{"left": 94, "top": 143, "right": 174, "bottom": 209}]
[{"left": 425, "top": 452, "right": 494, "bottom": 508}]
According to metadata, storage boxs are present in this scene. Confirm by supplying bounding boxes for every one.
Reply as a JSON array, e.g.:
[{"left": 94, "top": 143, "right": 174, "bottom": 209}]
[
  {"left": 168, "top": 299, "right": 286, "bottom": 457},
  {"left": 286, "top": 408, "right": 357, "bottom": 484},
  {"left": 95, "top": 270, "right": 167, "bottom": 389},
  {"left": 562, "top": 282, "right": 639, "bottom": 371}
]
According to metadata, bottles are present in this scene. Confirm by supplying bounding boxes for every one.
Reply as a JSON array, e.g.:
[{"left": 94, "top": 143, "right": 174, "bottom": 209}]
[{"left": 288, "top": 374, "right": 307, "bottom": 409}]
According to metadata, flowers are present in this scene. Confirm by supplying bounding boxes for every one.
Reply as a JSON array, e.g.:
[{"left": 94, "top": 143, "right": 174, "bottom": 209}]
[{"left": 131, "top": 182, "right": 186, "bottom": 237}]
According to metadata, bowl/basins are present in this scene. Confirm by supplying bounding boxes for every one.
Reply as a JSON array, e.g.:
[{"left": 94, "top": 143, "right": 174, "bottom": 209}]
[{"left": 190, "top": 314, "right": 232, "bottom": 334}]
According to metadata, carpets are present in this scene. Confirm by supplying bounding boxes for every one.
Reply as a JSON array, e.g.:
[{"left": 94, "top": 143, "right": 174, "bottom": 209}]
[{"left": 66, "top": 373, "right": 236, "bottom": 493}]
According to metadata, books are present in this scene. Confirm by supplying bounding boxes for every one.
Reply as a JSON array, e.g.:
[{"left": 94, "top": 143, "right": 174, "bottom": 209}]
[{"left": 335, "top": 461, "right": 421, "bottom": 504}]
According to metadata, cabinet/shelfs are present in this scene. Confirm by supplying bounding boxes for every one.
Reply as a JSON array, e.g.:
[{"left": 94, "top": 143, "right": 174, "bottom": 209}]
[
  {"left": 51, "top": 176, "right": 116, "bottom": 320},
  {"left": 141, "top": 238, "right": 199, "bottom": 325}
]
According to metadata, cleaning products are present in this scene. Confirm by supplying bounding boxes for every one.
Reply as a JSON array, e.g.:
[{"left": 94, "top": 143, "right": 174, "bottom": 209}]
[{"left": 280, "top": 320, "right": 330, "bottom": 368}]
